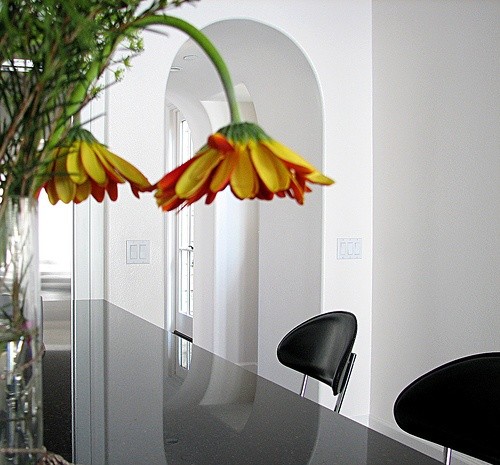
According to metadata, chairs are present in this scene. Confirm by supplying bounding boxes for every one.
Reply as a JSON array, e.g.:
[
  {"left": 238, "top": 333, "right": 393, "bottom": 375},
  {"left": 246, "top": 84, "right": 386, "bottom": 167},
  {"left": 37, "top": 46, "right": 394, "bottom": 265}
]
[
  {"left": 276, "top": 311, "right": 357, "bottom": 413},
  {"left": 393, "top": 351, "right": 500, "bottom": 465}
]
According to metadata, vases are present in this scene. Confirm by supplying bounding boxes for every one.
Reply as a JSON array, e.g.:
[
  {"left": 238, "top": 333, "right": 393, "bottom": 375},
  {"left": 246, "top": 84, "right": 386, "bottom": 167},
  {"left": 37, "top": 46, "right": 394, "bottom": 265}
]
[{"left": 1, "top": 196, "right": 44, "bottom": 465}]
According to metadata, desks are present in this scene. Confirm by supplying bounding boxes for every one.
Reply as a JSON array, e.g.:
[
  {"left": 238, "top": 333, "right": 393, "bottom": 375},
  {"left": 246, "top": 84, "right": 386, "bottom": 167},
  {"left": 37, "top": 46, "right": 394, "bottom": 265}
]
[{"left": 0, "top": 299, "right": 446, "bottom": 465}]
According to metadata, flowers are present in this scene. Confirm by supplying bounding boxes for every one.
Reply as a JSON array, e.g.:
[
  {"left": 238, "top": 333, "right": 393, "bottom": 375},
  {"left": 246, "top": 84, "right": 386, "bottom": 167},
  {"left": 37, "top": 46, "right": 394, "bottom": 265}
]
[{"left": 0, "top": 0, "right": 334, "bottom": 442}]
